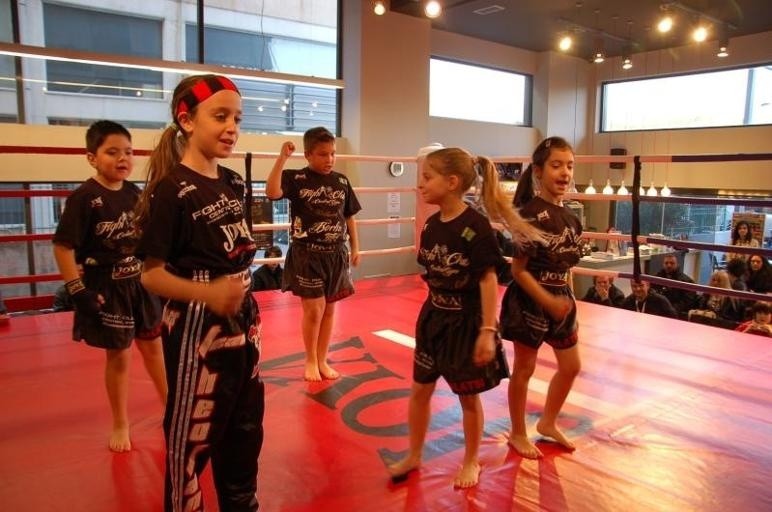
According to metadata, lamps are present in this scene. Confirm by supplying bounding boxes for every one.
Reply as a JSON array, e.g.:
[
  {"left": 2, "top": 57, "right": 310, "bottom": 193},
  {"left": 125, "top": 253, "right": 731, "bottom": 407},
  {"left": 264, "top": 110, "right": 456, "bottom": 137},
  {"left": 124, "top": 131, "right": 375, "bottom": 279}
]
[
  {"left": 617, "top": 19, "right": 635, "bottom": 196},
  {"left": 716, "top": 41, "right": 733, "bottom": 60},
  {"left": 661, "top": 29, "right": 674, "bottom": 198},
  {"left": 591, "top": 51, "right": 606, "bottom": 67},
  {"left": 622, "top": 57, "right": 634, "bottom": 72},
  {"left": 647, "top": 31, "right": 661, "bottom": 198},
  {"left": 603, "top": 15, "right": 620, "bottom": 195},
  {"left": 585, "top": 5, "right": 600, "bottom": 194},
  {"left": 638, "top": 28, "right": 651, "bottom": 195},
  {"left": 569, "top": 2, "right": 582, "bottom": 194}
]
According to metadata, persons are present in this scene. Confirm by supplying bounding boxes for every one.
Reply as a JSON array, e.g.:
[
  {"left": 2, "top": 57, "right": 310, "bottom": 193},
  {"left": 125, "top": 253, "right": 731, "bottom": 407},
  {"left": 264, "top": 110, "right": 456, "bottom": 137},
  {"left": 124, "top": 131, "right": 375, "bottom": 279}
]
[
  {"left": 266, "top": 126, "right": 362, "bottom": 383},
  {"left": 499, "top": 140, "right": 585, "bottom": 460},
  {"left": 733, "top": 300, "right": 772, "bottom": 337},
  {"left": 582, "top": 276, "right": 624, "bottom": 310},
  {"left": 252, "top": 246, "right": 287, "bottom": 291},
  {"left": 727, "top": 221, "right": 759, "bottom": 259},
  {"left": 746, "top": 253, "right": 772, "bottom": 297},
  {"left": 137, "top": 73, "right": 265, "bottom": 512},
  {"left": 54, "top": 262, "right": 84, "bottom": 313},
  {"left": 621, "top": 279, "right": 675, "bottom": 321},
  {"left": 52, "top": 119, "right": 172, "bottom": 455},
  {"left": 649, "top": 254, "right": 696, "bottom": 314},
  {"left": 683, "top": 271, "right": 738, "bottom": 319},
  {"left": 367, "top": 148, "right": 551, "bottom": 494},
  {"left": 722, "top": 258, "right": 746, "bottom": 322}
]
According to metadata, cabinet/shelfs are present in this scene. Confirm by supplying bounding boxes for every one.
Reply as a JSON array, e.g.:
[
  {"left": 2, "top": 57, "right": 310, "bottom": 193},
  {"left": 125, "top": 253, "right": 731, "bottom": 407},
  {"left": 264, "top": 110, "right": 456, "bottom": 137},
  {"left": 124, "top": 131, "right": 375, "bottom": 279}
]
[{"left": 572, "top": 240, "right": 688, "bottom": 300}]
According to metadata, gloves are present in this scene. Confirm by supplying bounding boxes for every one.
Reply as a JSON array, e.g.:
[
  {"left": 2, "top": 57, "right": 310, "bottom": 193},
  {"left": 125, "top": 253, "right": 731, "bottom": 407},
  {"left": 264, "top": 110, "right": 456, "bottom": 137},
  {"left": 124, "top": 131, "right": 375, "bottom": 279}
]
[{"left": 64, "top": 277, "right": 99, "bottom": 315}]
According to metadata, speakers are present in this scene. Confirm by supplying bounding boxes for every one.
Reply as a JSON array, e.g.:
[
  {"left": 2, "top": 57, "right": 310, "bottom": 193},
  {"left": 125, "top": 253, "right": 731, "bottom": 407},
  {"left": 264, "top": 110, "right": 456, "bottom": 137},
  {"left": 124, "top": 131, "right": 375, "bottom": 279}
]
[{"left": 609, "top": 147, "right": 627, "bottom": 170}]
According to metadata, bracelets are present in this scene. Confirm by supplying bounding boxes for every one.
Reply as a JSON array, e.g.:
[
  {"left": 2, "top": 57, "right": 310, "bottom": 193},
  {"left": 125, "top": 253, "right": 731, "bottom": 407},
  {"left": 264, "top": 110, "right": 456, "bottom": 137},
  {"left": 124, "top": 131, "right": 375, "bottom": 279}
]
[{"left": 479, "top": 326, "right": 499, "bottom": 334}]
